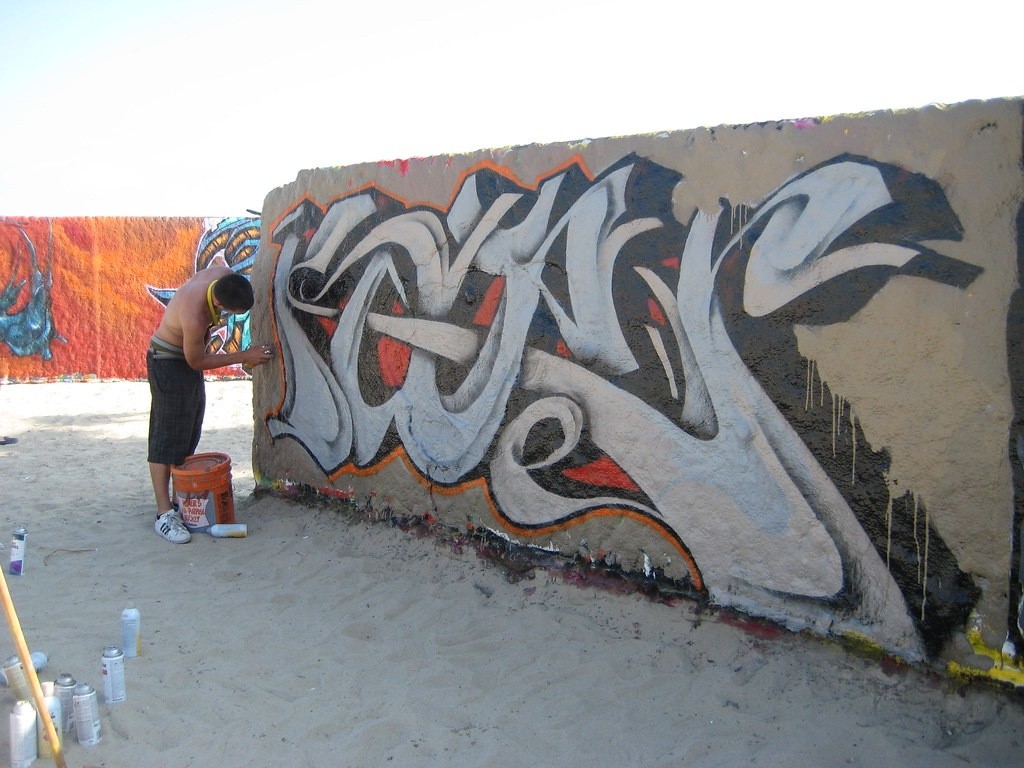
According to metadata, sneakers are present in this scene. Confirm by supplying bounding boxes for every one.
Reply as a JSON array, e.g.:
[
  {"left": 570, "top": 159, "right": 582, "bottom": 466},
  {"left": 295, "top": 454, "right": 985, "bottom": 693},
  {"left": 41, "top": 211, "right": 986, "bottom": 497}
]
[
  {"left": 171, "top": 502, "right": 180, "bottom": 512},
  {"left": 154, "top": 509, "right": 192, "bottom": 544}
]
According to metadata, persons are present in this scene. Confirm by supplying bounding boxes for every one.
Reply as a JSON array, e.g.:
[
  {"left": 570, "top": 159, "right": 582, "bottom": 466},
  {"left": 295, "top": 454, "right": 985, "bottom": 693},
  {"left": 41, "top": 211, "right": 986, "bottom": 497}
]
[{"left": 146, "top": 265, "right": 273, "bottom": 543}]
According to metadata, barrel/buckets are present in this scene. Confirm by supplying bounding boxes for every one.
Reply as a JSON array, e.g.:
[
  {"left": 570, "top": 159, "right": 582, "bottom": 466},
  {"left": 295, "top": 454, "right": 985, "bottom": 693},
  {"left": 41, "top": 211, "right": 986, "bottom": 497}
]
[{"left": 170, "top": 452, "right": 234, "bottom": 534}]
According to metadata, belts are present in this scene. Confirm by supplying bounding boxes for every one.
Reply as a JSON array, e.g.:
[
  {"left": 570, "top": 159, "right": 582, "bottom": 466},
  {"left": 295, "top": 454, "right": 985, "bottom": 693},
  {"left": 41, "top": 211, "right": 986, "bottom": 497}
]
[{"left": 148, "top": 347, "right": 185, "bottom": 359}]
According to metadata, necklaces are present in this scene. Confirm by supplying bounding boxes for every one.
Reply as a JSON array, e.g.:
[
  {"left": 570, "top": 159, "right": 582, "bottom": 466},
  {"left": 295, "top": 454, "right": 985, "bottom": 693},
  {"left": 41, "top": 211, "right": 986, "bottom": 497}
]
[{"left": 207, "top": 280, "right": 219, "bottom": 326}]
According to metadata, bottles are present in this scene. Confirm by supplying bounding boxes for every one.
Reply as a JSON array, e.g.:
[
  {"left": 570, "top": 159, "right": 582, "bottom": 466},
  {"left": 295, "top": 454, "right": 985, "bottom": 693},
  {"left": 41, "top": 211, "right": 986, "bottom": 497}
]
[
  {"left": 207, "top": 524, "right": 249, "bottom": 538},
  {"left": 1, "top": 653, "right": 106, "bottom": 767},
  {"left": 99, "top": 646, "right": 130, "bottom": 704},
  {"left": 245, "top": 346, "right": 274, "bottom": 370},
  {"left": 9, "top": 525, "right": 30, "bottom": 576},
  {"left": 119, "top": 602, "right": 144, "bottom": 661}
]
